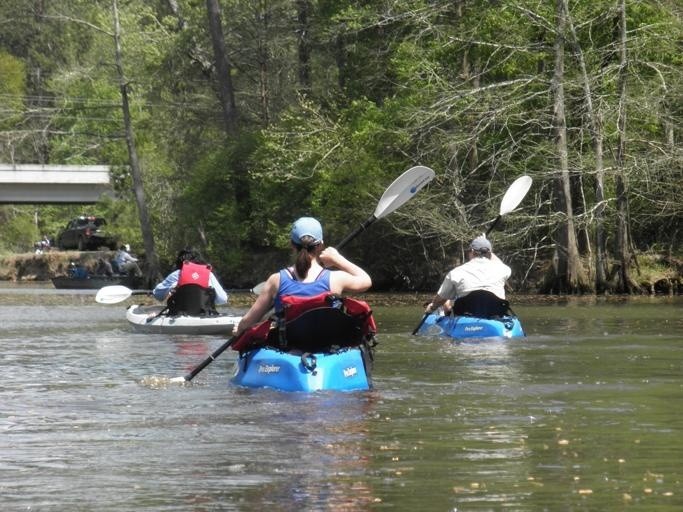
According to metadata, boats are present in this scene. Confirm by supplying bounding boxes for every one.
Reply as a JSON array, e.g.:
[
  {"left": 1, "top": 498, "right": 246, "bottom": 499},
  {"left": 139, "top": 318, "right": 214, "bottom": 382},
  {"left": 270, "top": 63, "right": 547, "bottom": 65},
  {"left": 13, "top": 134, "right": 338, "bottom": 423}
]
[
  {"left": 418, "top": 312, "right": 526, "bottom": 341},
  {"left": 230, "top": 345, "right": 370, "bottom": 395},
  {"left": 51, "top": 274, "right": 139, "bottom": 290},
  {"left": 125, "top": 304, "right": 242, "bottom": 335}
]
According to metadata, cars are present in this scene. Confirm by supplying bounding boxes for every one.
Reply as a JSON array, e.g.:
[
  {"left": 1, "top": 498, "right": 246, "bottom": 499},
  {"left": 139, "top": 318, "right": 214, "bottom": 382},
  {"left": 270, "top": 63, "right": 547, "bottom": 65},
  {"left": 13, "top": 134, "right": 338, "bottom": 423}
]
[{"left": 57, "top": 216, "right": 117, "bottom": 251}]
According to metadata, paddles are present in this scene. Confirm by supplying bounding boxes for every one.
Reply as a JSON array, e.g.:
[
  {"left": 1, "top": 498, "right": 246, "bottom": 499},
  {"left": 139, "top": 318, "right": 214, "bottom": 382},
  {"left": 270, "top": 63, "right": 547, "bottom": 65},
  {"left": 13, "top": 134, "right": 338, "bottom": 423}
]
[
  {"left": 95, "top": 285, "right": 153, "bottom": 305},
  {"left": 411, "top": 176, "right": 533, "bottom": 336},
  {"left": 184, "top": 165, "right": 435, "bottom": 378}
]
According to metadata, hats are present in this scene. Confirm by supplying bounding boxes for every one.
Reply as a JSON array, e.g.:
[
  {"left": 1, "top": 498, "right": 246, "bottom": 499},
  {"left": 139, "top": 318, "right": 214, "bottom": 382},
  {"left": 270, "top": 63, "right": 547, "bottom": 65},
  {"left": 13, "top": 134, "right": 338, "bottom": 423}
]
[
  {"left": 290, "top": 216, "right": 323, "bottom": 247},
  {"left": 462, "top": 236, "right": 491, "bottom": 253}
]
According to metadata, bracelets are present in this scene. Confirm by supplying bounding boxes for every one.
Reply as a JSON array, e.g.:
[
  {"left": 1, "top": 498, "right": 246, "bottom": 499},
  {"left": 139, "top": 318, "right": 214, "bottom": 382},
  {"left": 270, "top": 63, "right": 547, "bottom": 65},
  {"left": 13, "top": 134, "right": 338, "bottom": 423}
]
[{"left": 429, "top": 302, "right": 436, "bottom": 311}]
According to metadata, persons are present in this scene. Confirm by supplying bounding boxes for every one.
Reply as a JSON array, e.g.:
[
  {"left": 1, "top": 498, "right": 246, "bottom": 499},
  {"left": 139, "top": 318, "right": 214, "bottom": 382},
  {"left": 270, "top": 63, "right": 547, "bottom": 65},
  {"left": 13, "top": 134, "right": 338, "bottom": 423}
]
[
  {"left": 113, "top": 242, "right": 146, "bottom": 279},
  {"left": 65, "top": 253, "right": 93, "bottom": 280},
  {"left": 152, "top": 245, "right": 229, "bottom": 315},
  {"left": 34, "top": 234, "right": 50, "bottom": 254},
  {"left": 424, "top": 232, "right": 512, "bottom": 316},
  {"left": 92, "top": 249, "right": 116, "bottom": 280},
  {"left": 230, "top": 216, "right": 372, "bottom": 341}
]
